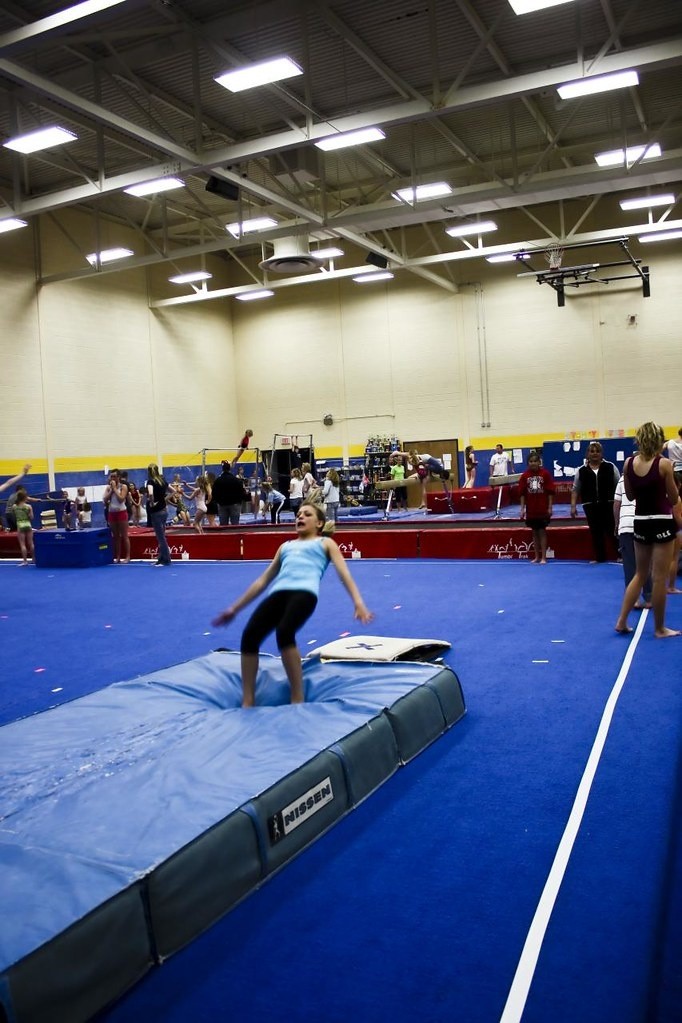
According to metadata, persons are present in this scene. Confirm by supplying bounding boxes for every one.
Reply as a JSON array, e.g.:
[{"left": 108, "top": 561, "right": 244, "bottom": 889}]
[
  {"left": 320, "top": 469, "right": 340, "bottom": 523},
  {"left": 570, "top": 443, "right": 623, "bottom": 563},
  {"left": 230, "top": 429, "right": 253, "bottom": 468},
  {"left": 519, "top": 453, "right": 553, "bottom": 563},
  {"left": 167, "top": 460, "right": 286, "bottom": 534},
  {"left": 102, "top": 464, "right": 171, "bottom": 566},
  {"left": 5, "top": 485, "right": 42, "bottom": 566},
  {"left": 390, "top": 451, "right": 449, "bottom": 509},
  {"left": 213, "top": 504, "right": 374, "bottom": 708},
  {"left": 47, "top": 488, "right": 92, "bottom": 530},
  {"left": 489, "top": 444, "right": 515, "bottom": 477},
  {"left": 288, "top": 435, "right": 316, "bottom": 516},
  {"left": 0, "top": 464, "right": 32, "bottom": 492},
  {"left": 614, "top": 422, "right": 682, "bottom": 638},
  {"left": 463, "top": 446, "right": 478, "bottom": 488}
]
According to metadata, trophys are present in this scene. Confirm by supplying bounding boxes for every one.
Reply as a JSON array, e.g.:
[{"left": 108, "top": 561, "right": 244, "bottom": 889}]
[{"left": 364, "top": 435, "right": 401, "bottom": 482}]
